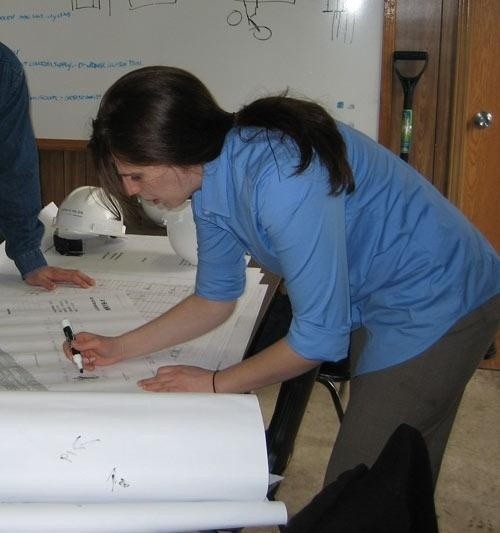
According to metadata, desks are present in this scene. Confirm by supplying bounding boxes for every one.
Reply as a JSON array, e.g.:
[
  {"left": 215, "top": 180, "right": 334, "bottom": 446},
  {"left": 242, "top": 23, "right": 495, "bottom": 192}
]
[{"left": 0, "top": 205, "right": 284, "bottom": 533}]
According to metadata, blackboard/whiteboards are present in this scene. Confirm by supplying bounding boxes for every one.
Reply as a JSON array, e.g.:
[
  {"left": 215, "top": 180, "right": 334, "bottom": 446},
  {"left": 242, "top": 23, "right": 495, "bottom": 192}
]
[{"left": 0, "top": 1, "right": 396, "bottom": 145}]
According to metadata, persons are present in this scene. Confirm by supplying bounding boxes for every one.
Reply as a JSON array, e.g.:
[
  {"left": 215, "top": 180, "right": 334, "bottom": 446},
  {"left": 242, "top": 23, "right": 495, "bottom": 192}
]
[
  {"left": 0, "top": 40, "right": 97, "bottom": 291},
  {"left": 61, "top": 64, "right": 499, "bottom": 533}
]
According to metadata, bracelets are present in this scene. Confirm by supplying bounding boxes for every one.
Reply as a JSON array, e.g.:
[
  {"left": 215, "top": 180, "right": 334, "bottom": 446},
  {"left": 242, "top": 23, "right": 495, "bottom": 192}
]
[{"left": 210, "top": 368, "right": 221, "bottom": 393}]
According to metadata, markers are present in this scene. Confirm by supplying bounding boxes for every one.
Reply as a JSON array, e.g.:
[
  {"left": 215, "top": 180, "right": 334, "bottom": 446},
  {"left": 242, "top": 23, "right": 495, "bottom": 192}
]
[{"left": 61, "top": 319, "right": 84, "bottom": 373}]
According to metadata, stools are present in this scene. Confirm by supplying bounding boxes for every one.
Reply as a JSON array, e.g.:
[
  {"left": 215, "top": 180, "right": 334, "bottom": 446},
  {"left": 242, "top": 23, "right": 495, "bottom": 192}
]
[{"left": 241, "top": 288, "right": 351, "bottom": 426}]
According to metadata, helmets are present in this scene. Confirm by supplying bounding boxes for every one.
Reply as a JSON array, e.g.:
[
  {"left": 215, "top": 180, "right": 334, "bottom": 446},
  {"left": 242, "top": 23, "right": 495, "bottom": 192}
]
[
  {"left": 166, "top": 199, "right": 251, "bottom": 266},
  {"left": 137, "top": 196, "right": 166, "bottom": 227},
  {"left": 54, "top": 187, "right": 126, "bottom": 255}
]
